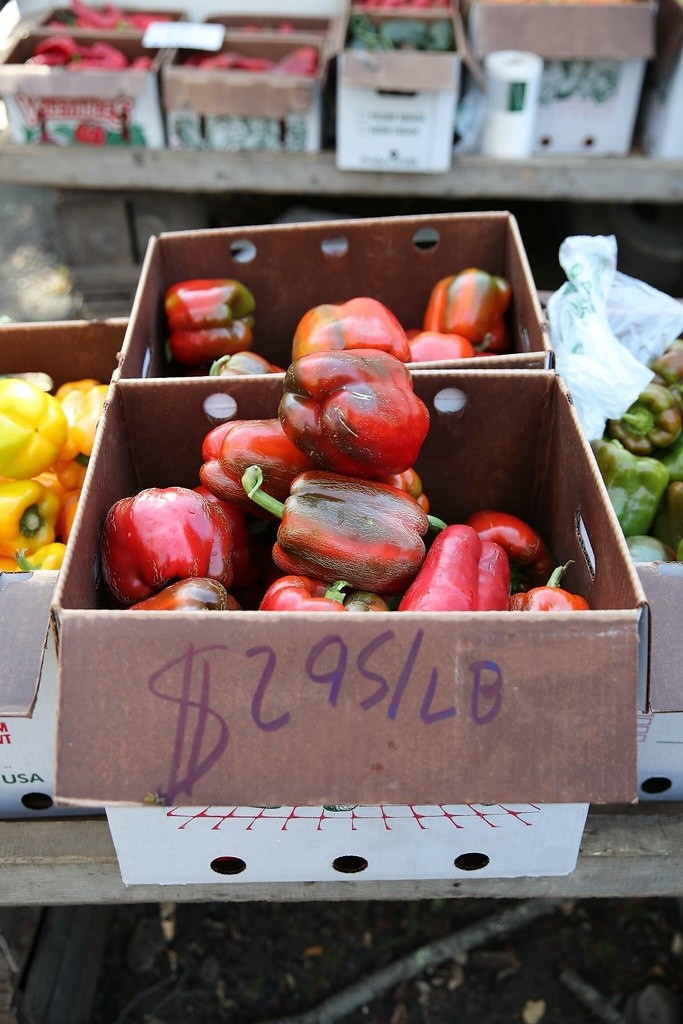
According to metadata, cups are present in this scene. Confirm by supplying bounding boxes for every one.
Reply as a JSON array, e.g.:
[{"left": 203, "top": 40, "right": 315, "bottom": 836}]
[{"left": 482, "top": 46, "right": 542, "bottom": 162}]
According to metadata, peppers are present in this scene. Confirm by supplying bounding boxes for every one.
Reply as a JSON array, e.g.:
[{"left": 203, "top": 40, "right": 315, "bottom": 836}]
[
  {"left": 22, "top": 2, "right": 454, "bottom": 65},
  {"left": 1, "top": 262, "right": 683, "bottom": 612}
]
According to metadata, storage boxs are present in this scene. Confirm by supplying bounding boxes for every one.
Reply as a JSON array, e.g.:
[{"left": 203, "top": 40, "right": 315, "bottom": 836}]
[
  {"left": 0, "top": 319, "right": 131, "bottom": 820},
  {"left": 52, "top": 211, "right": 654, "bottom": 887},
  {"left": 538, "top": 300, "right": 682, "bottom": 805},
  {"left": 0, "top": 27, "right": 169, "bottom": 147},
  {"left": 200, "top": 12, "right": 332, "bottom": 40},
  {"left": 314, "top": 7, "right": 484, "bottom": 173},
  {"left": 468, "top": 1, "right": 656, "bottom": 160},
  {"left": 35, "top": 6, "right": 188, "bottom": 31},
  {"left": 160, "top": 36, "right": 330, "bottom": 152},
  {"left": 641, "top": 0, "right": 681, "bottom": 162}
]
